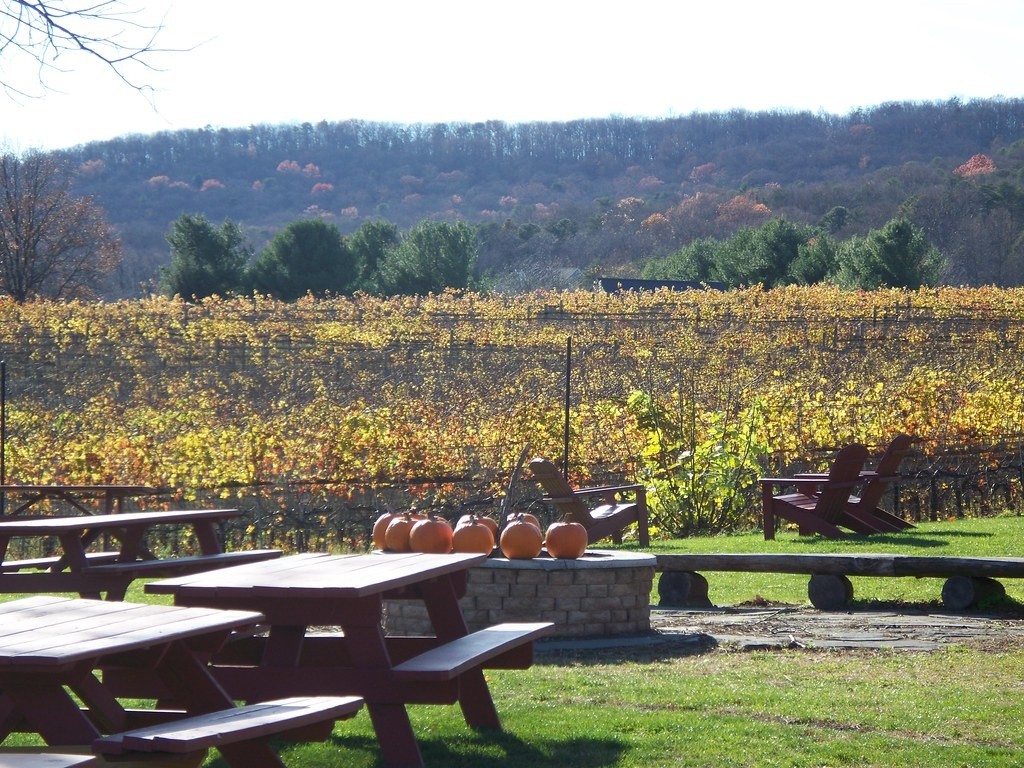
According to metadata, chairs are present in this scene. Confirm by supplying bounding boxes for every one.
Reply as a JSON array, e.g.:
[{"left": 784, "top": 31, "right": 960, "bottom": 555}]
[
  {"left": 757, "top": 434, "right": 918, "bottom": 541},
  {"left": 528, "top": 456, "right": 650, "bottom": 548}
]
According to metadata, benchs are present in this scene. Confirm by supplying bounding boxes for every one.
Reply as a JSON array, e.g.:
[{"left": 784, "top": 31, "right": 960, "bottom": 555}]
[
  {"left": 653, "top": 554, "right": 1024, "bottom": 610},
  {"left": 0, "top": 550, "right": 556, "bottom": 768}
]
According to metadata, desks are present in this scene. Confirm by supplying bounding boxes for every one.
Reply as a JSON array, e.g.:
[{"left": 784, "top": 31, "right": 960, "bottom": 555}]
[
  {"left": 0, "top": 595, "right": 286, "bottom": 768},
  {"left": 0, "top": 485, "right": 165, "bottom": 515},
  {"left": 0, "top": 509, "right": 240, "bottom": 600},
  {"left": 143, "top": 552, "right": 500, "bottom": 768}
]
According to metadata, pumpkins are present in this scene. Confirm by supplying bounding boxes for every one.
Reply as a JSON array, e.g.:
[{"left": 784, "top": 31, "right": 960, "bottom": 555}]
[{"left": 372, "top": 505, "right": 588, "bottom": 559}]
